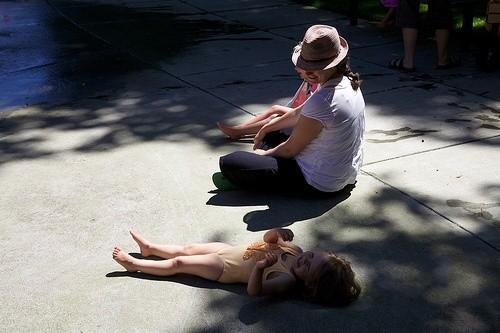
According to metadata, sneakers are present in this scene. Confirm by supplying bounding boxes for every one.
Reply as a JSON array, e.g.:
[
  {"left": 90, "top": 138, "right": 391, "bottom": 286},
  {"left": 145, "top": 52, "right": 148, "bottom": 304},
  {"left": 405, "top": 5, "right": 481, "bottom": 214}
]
[{"left": 213, "top": 172, "right": 240, "bottom": 189}]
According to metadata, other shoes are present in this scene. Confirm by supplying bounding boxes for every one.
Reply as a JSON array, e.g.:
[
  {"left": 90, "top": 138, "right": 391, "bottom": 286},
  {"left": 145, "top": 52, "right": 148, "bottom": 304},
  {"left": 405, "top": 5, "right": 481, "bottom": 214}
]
[{"left": 377, "top": 21, "right": 385, "bottom": 28}]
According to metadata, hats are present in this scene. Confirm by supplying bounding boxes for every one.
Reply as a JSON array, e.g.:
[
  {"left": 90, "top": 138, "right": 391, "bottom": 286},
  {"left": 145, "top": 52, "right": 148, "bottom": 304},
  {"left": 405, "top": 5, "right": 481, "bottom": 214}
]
[{"left": 292, "top": 24, "right": 348, "bottom": 71}]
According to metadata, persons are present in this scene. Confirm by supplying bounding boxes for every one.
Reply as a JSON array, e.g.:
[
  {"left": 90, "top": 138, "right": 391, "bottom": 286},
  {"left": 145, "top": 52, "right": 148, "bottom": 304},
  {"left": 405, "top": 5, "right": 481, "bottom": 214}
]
[
  {"left": 112, "top": 228, "right": 363, "bottom": 308},
  {"left": 375, "top": 0, "right": 464, "bottom": 72},
  {"left": 216, "top": 24, "right": 366, "bottom": 202}
]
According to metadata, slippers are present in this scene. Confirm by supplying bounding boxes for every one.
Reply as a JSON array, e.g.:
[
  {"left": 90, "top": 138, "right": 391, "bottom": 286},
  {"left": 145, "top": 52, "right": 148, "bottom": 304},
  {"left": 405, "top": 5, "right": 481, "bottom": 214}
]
[
  {"left": 389, "top": 58, "right": 413, "bottom": 72},
  {"left": 437, "top": 55, "right": 462, "bottom": 69}
]
